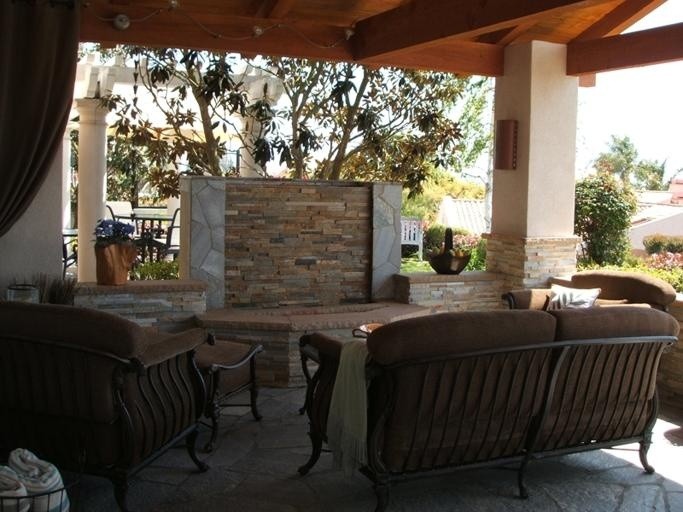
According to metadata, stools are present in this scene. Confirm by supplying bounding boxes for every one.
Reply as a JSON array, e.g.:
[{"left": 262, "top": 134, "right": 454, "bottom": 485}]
[{"left": 195, "top": 335, "right": 264, "bottom": 453}]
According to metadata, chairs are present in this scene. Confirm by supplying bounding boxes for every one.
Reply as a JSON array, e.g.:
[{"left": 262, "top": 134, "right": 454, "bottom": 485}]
[
  {"left": 501, "top": 270, "right": 676, "bottom": 313},
  {"left": 63, "top": 199, "right": 182, "bottom": 277},
  {"left": 0, "top": 300, "right": 216, "bottom": 512},
  {"left": 400, "top": 215, "right": 425, "bottom": 262}
]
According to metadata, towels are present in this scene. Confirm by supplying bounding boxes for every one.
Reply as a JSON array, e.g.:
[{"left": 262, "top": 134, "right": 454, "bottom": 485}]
[{"left": 0, "top": 448, "right": 71, "bottom": 512}]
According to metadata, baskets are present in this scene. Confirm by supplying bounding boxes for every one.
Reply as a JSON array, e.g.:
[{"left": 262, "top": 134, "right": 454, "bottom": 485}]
[{"left": 1, "top": 474, "right": 74, "bottom": 512}]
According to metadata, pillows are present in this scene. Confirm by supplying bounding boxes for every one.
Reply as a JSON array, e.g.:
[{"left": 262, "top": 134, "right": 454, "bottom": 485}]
[{"left": 545, "top": 283, "right": 602, "bottom": 311}]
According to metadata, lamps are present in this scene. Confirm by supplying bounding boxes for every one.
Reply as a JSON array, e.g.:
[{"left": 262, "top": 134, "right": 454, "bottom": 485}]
[
  {"left": 7, "top": 284, "right": 40, "bottom": 306},
  {"left": 494, "top": 119, "right": 518, "bottom": 170}
]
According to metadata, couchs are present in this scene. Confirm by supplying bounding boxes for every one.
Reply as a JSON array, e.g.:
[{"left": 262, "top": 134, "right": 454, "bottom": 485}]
[{"left": 297, "top": 306, "right": 680, "bottom": 512}]
